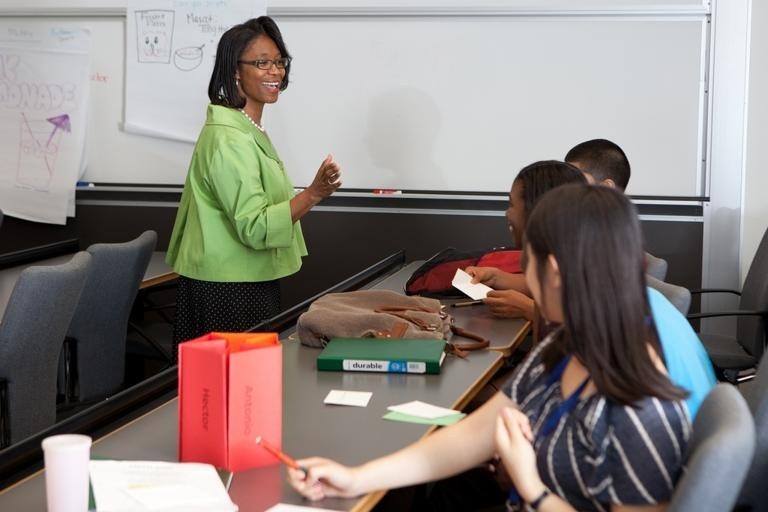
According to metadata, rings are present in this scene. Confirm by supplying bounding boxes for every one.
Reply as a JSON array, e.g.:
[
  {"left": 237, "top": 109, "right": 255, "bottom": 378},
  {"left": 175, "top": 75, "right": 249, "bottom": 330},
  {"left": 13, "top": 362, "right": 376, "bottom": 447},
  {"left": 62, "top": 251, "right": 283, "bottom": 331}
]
[{"left": 328, "top": 179, "right": 332, "bottom": 185}]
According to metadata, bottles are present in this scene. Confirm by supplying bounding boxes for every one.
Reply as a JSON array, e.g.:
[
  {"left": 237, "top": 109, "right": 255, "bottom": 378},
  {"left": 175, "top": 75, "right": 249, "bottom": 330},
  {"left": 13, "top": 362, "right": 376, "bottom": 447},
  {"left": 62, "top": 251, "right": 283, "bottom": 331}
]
[{"left": 41, "top": 433, "right": 93, "bottom": 512}]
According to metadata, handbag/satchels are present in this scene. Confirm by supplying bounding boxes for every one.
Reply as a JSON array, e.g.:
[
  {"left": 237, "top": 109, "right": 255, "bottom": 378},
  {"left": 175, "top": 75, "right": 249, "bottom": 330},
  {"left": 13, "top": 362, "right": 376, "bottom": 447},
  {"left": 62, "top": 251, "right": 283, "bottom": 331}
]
[
  {"left": 297, "top": 290, "right": 454, "bottom": 348},
  {"left": 404, "top": 246, "right": 526, "bottom": 299}
]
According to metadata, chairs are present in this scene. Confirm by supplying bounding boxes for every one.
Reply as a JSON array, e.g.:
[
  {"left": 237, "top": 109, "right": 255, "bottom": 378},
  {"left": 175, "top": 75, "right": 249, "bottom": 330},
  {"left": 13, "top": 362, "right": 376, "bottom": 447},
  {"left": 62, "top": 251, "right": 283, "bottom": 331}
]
[
  {"left": 57, "top": 230, "right": 159, "bottom": 409},
  {"left": 0, "top": 250, "right": 94, "bottom": 447},
  {"left": 629, "top": 222, "right": 768, "bottom": 511}
]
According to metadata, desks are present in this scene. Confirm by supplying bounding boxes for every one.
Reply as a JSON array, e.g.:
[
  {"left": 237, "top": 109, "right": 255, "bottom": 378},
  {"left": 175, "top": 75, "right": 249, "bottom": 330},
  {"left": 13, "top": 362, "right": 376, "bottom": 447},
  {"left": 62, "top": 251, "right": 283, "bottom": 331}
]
[
  {"left": 0, "top": 250, "right": 181, "bottom": 407},
  {"left": 2, "top": 262, "right": 534, "bottom": 512}
]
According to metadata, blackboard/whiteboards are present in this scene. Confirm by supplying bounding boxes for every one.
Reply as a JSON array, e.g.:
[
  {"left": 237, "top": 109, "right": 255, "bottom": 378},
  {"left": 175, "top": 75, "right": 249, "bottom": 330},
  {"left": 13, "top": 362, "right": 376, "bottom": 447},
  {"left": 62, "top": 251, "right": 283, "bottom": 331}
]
[{"left": 0, "top": 2, "right": 712, "bottom": 203}]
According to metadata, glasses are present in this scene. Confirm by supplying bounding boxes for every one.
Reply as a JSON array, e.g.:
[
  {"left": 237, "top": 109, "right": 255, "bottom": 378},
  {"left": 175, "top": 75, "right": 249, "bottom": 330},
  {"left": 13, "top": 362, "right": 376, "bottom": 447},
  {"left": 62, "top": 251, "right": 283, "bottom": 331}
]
[{"left": 237, "top": 58, "right": 289, "bottom": 70}]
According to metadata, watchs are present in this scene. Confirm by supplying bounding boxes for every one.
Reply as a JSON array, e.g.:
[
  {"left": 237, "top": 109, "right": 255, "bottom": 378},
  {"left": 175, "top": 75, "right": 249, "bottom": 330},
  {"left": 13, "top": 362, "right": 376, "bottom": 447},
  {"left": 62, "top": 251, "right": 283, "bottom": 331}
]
[{"left": 523, "top": 486, "right": 552, "bottom": 512}]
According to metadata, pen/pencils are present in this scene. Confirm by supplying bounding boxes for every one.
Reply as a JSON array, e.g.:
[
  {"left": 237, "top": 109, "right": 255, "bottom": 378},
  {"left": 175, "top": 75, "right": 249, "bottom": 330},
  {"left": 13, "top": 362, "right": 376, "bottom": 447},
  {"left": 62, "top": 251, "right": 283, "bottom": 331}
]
[
  {"left": 371, "top": 188, "right": 403, "bottom": 196},
  {"left": 76, "top": 182, "right": 94, "bottom": 188},
  {"left": 255, "top": 436, "right": 308, "bottom": 474},
  {"left": 451, "top": 299, "right": 481, "bottom": 308}
]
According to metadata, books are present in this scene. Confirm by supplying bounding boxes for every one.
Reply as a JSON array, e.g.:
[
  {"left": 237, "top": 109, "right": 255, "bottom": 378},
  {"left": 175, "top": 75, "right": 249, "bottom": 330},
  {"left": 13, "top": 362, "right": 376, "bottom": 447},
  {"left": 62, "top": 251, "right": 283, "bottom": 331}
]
[{"left": 315, "top": 335, "right": 447, "bottom": 375}]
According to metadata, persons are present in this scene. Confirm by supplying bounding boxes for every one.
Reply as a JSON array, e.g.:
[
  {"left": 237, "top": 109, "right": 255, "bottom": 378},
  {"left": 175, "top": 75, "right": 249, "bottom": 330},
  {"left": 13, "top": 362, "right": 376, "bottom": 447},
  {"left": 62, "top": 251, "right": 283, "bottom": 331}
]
[{"left": 166, "top": 13, "right": 343, "bottom": 365}]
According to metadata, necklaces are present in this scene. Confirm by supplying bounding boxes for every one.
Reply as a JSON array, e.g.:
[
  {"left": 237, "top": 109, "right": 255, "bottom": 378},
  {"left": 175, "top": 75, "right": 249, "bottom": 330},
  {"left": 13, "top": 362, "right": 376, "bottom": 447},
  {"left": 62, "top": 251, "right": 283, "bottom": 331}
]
[{"left": 238, "top": 107, "right": 266, "bottom": 132}]
